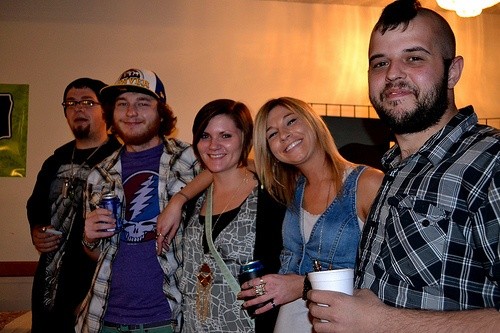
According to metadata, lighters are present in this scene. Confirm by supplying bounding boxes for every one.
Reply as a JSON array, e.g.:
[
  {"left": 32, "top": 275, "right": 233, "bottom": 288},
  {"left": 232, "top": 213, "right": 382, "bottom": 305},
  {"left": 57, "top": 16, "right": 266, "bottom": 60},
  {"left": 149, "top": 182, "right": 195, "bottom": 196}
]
[{"left": 39, "top": 226, "right": 62, "bottom": 234}]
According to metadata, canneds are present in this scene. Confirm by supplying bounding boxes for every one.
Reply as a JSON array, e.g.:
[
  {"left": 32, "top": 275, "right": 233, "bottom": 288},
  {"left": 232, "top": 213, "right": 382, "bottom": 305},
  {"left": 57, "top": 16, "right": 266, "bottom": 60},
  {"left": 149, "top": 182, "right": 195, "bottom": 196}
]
[
  {"left": 239, "top": 260, "right": 263, "bottom": 274},
  {"left": 99, "top": 194, "right": 123, "bottom": 234}
]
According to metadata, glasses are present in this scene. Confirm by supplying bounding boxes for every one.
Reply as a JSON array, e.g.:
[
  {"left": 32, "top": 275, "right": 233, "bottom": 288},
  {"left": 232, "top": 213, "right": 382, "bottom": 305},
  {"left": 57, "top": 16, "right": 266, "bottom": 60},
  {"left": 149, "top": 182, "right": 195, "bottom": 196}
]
[{"left": 62, "top": 100, "right": 100, "bottom": 108}]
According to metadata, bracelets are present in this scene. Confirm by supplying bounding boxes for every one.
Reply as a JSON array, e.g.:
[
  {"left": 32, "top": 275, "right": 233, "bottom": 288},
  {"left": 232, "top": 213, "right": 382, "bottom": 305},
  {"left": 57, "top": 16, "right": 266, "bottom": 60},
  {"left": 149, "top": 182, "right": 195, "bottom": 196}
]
[
  {"left": 302, "top": 273, "right": 312, "bottom": 301},
  {"left": 178, "top": 192, "right": 190, "bottom": 201},
  {"left": 83, "top": 238, "right": 100, "bottom": 251}
]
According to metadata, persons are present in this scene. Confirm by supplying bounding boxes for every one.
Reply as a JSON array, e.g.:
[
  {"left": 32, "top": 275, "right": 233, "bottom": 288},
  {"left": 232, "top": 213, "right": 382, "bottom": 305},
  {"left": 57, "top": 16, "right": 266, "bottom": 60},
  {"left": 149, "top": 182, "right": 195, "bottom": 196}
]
[
  {"left": 75, "top": 69, "right": 205, "bottom": 333},
  {"left": 27, "top": 78, "right": 122, "bottom": 333},
  {"left": 307, "top": 0, "right": 500, "bottom": 333},
  {"left": 157, "top": 97, "right": 385, "bottom": 333},
  {"left": 181, "top": 99, "right": 288, "bottom": 333}
]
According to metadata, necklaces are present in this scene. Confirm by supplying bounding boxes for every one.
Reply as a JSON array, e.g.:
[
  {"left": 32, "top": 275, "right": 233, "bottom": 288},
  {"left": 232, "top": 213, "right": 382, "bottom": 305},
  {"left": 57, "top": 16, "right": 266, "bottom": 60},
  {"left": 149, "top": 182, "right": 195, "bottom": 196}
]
[
  {"left": 66, "top": 145, "right": 102, "bottom": 196},
  {"left": 195, "top": 172, "right": 248, "bottom": 314},
  {"left": 314, "top": 180, "right": 333, "bottom": 272}
]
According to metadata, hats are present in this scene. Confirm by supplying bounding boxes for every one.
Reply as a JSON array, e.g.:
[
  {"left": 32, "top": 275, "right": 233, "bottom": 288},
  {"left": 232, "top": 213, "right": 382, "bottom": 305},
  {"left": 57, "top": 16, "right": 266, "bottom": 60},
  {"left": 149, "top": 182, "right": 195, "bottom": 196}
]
[{"left": 99, "top": 67, "right": 166, "bottom": 104}]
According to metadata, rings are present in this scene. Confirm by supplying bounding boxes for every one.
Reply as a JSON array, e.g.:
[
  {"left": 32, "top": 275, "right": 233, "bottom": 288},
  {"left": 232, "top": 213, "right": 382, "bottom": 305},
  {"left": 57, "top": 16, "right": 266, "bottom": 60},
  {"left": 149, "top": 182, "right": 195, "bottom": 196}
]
[
  {"left": 271, "top": 300, "right": 276, "bottom": 307},
  {"left": 160, "top": 234, "right": 165, "bottom": 238},
  {"left": 260, "top": 278, "right": 265, "bottom": 283},
  {"left": 247, "top": 280, "right": 253, "bottom": 287},
  {"left": 255, "top": 284, "right": 266, "bottom": 295}
]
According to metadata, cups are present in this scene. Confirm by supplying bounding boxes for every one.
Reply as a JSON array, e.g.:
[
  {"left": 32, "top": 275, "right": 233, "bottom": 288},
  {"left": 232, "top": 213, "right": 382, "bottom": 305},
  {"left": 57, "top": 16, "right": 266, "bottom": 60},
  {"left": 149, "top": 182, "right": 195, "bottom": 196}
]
[
  {"left": 238, "top": 267, "right": 274, "bottom": 319},
  {"left": 306, "top": 269, "right": 355, "bottom": 322}
]
